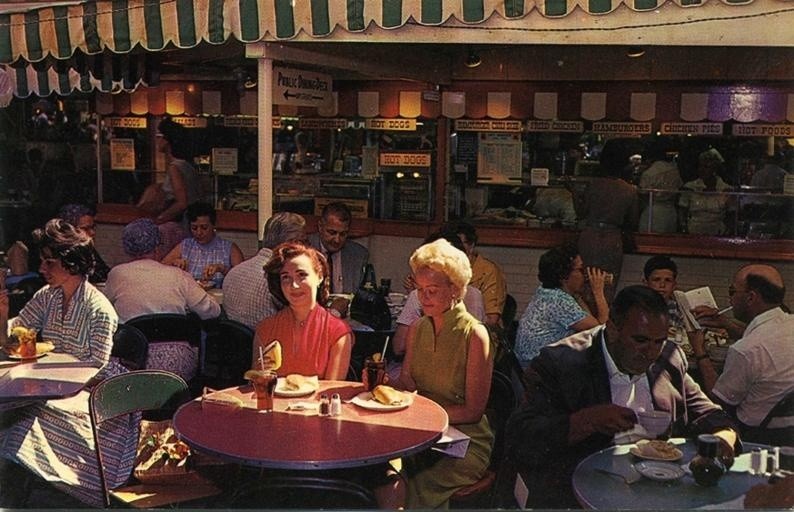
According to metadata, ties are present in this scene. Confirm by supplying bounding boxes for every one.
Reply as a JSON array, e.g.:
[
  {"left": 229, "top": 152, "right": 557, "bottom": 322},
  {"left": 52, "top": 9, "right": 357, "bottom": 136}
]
[{"left": 326, "top": 251, "right": 337, "bottom": 296}]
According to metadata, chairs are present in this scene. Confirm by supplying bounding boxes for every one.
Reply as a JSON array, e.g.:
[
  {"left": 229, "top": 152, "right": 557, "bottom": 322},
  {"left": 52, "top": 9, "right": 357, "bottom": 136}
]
[
  {"left": 214, "top": 319, "right": 255, "bottom": 368},
  {"left": 450, "top": 370, "right": 517, "bottom": 510},
  {"left": 112, "top": 323, "right": 150, "bottom": 370},
  {"left": 124, "top": 312, "right": 204, "bottom": 413},
  {"left": 501, "top": 293, "right": 518, "bottom": 334},
  {"left": 498, "top": 333, "right": 528, "bottom": 392},
  {"left": 748, "top": 390, "right": 794, "bottom": 442},
  {"left": 87, "top": 368, "right": 222, "bottom": 509}
]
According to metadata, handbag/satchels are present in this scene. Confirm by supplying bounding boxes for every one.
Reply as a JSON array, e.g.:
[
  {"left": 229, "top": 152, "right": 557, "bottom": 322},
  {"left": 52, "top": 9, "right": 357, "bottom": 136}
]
[{"left": 349, "top": 264, "right": 393, "bottom": 332}]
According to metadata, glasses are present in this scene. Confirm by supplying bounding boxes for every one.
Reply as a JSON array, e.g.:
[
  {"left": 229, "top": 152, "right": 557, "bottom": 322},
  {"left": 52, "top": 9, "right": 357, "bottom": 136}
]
[{"left": 726, "top": 284, "right": 749, "bottom": 296}]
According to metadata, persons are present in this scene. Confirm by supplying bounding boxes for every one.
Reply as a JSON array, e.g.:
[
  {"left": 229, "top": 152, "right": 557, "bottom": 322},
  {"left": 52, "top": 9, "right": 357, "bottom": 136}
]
[
  {"left": 742, "top": 144, "right": 790, "bottom": 238},
  {"left": 677, "top": 150, "right": 735, "bottom": 237},
  {"left": 572, "top": 137, "right": 638, "bottom": 314},
  {"left": 685, "top": 263, "right": 790, "bottom": 444},
  {"left": 508, "top": 283, "right": 742, "bottom": 472},
  {"left": 103, "top": 217, "right": 220, "bottom": 384},
  {"left": 0, "top": 218, "right": 140, "bottom": 511},
  {"left": 157, "top": 140, "right": 202, "bottom": 242},
  {"left": 221, "top": 211, "right": 307, "bottom": 331},
  {"left": 638, "top": 133, "right": 680, "bottom": 233},
  {"left": 309, "top": 201, "right": 375, "bottom": 311},
  {"left": 59, "top": 201, "right": 113, "bottom": 277},
  {"left": 161, "top": 203, "right": 244, "bottom": 292},
  {"left": 394, "top": 231, "right": 486, "bottom": 355},
  {"left": 513, "top": 241, "right": 611, "bottom": 368},
  {"left": 642, "top": 253, "right": 686, "bottom": 338},
  {"left": 455, "top": 218, "right": 505, "bottom": 328},
  {"left": 361, "top": 238, "right": 495, "bottom": 512},
  {"left": 250, "top": 241, "right": 355, "bottom": 384}
]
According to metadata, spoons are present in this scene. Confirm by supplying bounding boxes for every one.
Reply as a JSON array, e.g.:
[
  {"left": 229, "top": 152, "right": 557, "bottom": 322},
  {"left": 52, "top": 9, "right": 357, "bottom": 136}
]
[{"left": 594, "top": 468, "right": 642, "bottom": 485}]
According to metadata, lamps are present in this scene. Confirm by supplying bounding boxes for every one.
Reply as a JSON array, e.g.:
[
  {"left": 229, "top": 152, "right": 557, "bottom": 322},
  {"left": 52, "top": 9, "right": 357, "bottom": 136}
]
[
  {"left": 462, "top": 53, "right": 483, "bottom": 68},
  {"left": 626, "top": 47, "right": 646, "bottom": 58}
]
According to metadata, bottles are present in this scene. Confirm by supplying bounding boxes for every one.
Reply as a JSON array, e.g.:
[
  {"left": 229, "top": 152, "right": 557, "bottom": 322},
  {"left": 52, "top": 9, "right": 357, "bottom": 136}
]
[
  {"left": 318, "top": 393, "right": 330, "bottom": 417},
  {"left": 330, "top": 393, "right": 341, "bottom": 417}
]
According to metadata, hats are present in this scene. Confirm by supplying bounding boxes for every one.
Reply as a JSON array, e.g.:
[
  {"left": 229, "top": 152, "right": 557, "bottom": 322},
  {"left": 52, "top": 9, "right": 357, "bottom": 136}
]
[{"left": 123, "top": 218, "right": 166, "bottom": 254}]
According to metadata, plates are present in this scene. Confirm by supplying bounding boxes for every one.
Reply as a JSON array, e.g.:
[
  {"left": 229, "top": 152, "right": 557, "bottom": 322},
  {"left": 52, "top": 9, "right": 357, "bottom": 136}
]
[
  {"left": 194, "top": 278, "right": 217, "bottom": 292},
  {"left": 351, "top": 390, "right": 414, "bottom": 412},
  {"left": 387, "top": 303, "right": 404, "bottom": 306},
  {"left": 96, "top": 282, "right": 105, "bottom": 287},
  {"left": 8, "top": 351, "right": 47, "bottom": 360},
  {"left": 274, "top": 376, "right": 318, "bottom": 398},
  {"left": 636, "top": 460, "right": 685, "bottom": 482},
  {"left": 630, "top": 445, "right": 683, "bottom": 461}
]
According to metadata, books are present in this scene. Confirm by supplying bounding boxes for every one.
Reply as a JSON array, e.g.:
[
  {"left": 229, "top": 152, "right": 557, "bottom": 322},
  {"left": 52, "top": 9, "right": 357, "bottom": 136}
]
[{"left": 672, "top": 282, "right": 723, "bottom": 335}]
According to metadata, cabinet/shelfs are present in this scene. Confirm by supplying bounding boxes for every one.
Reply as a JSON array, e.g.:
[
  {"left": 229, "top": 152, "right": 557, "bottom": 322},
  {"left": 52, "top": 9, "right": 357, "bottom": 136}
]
[
  {"left": 447, "top": 176, "right": 794, "bottom": 261},
  {"left": 312, "top": 174, "right": 376, "bottom": 219},
  {"left": 378, "top": 148, "right": 436, "bottom": 223}
]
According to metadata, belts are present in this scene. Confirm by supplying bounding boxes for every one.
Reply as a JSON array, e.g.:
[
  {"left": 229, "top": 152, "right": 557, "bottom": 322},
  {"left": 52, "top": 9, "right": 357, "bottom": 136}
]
[{"left": 585, "top": 220, "right": 622, "bottom": 231}]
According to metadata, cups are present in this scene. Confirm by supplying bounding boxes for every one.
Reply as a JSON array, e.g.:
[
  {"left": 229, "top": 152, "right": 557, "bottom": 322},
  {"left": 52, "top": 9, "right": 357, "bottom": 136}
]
[
  {"left": 584, "top": 273, "right": 613, "bottom": 286},
  {"left": 389, "top": 293, "right": 404, "bottom": 303},
  {"left": 709, "top": 344, "right": 729, "bottom": 362},
  {"left": 19, "top": 333, "right": 37, "bottom": 364},
  {"left": 252, "top": 376, "right": 277, "bottom": 418},
  {"left": 634, "top": 411, "right": 672, "bottom": 435},
  {"left": 364, "top": 358, "right": 387, "bottom": 391}
]
